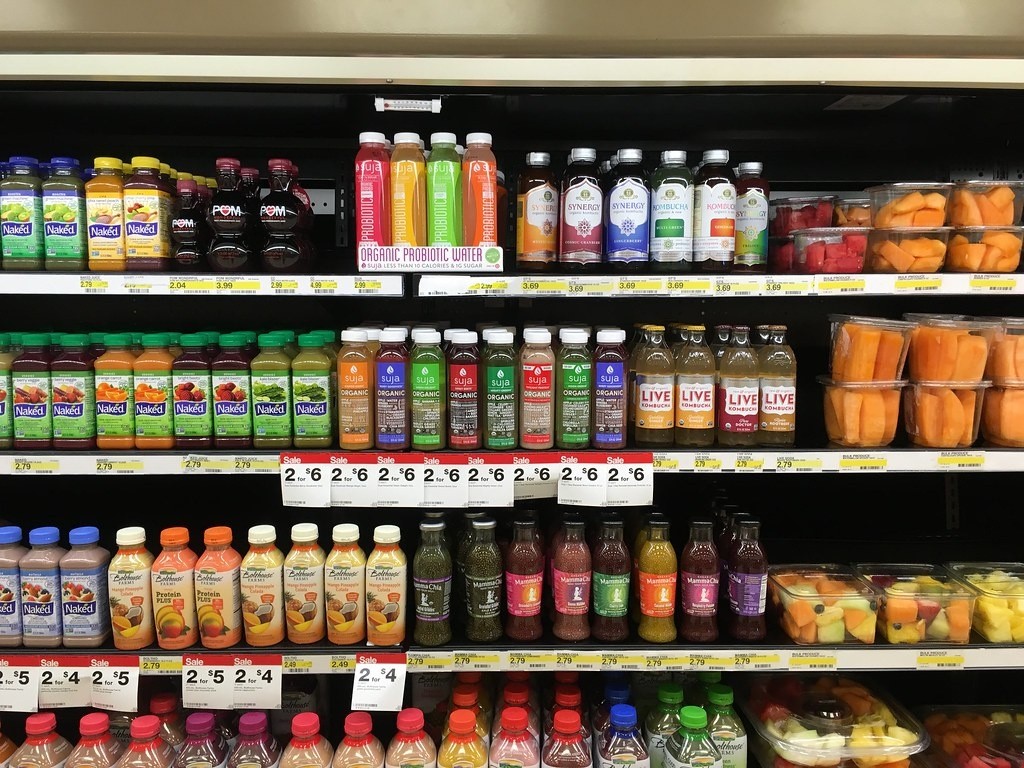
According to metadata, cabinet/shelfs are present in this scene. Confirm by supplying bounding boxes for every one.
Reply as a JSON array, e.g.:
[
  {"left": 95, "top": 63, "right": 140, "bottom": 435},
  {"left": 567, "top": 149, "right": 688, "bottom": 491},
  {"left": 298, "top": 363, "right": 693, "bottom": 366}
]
[{"left": 0, "top": 53, "right": 1024, "bottom": 676}]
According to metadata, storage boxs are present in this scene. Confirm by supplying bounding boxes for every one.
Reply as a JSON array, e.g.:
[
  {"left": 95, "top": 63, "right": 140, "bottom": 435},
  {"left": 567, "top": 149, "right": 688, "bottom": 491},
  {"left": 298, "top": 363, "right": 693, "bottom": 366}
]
[
  {"left": 910, "top": 703, "right": 1024, "bottom": 768},
  {"left": 941, "top": 561, "right": 1024, "bottom": 645},
  {"left": 769, "top": 180, "right": 1024, "bottom": 274},
  {"left": 817, "top": 311, "right": 1024, "bottom": 450},
  {"left": 767, "top": 563, "right": 884, "bottom": 647},
  {"left": 734, "top": 673, "right": 931, "bottom": 768},
  {"left": 856, "top": 563, "right": 984, "bottom": 646}
]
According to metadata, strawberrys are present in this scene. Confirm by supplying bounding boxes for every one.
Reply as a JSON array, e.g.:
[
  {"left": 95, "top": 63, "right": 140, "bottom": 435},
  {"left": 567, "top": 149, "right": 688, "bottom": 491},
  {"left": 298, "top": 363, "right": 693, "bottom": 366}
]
[{"left": 746, "top": 677, "right": 806, "bottom": 724}]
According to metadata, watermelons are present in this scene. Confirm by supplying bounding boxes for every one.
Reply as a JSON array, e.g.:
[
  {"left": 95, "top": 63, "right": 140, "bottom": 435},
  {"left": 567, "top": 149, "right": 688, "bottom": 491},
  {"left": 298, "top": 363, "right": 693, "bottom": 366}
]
[
  {"left": 770, "top": 199, "right": 865, "bottom": 271},
  {"left": 948, "top": 742, "right": 1024, "bottom": 768}
]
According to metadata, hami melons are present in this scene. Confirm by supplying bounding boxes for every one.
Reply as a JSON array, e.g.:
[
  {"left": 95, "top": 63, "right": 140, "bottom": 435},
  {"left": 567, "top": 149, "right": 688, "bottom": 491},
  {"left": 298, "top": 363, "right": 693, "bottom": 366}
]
[
  {"left": 772, "top": 574, "right": 975, "bottom": 642},
  {"left": 824, "top": 324, "right": 1024, "bottom": 449},
  {"left": 923, "top": 711, "right": 991, "bottom": 754},
  {"left": 833, "top": 187, "right": 1023, "bottom": 273}
]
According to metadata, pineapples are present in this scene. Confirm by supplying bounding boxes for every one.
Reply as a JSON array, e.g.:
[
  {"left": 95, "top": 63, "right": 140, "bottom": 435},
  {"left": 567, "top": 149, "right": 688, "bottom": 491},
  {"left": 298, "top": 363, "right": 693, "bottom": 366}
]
[
  {"left": 846, "top": 702, "right": 921, "bottom": 768},
  {"left": 957, "top": 576, "right": 1024, "bottom": 644}
]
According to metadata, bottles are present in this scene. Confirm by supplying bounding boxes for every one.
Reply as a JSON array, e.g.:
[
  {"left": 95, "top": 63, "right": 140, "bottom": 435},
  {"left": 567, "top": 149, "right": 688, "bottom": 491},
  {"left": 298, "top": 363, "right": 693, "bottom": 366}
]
[
  {"left": 171, "top": 331, "right": 257, "bottom": 448},
  {"left": 516, "top": 151, "right": 559, "bottom": 276},
  {"left": 208, "top": 159, "right": 260, "bottom": 274},
  {"left": 731, "top": 161, "right": 771, "bottom": 275},
  {"left": 341, "top": 323, "right": 632, "bottom": 452},
  {"left": 12, "top": 332, "right": 106, "bottom": 451},
  {"left": 412, "top": 506, "right": 759, "bottom": 652},
  {"left": 0, "top": 331, "right": 29, "bottom": 452},
  {"left": 251, "top": 331, "right": 339, "bottom": 448},
  {"left": 600, "top": 149, "right": 650, "bottom": 275},
  {"left": 0, "top": 527, "right": 111, "bottom": 648},
  {"left": 239, "top": 523, "right": 408, "bottom": 647},
  {"left": 0, "top": 671, "right": 748, "bottom": 768},
  {"left": 259, "top": 159, "right": 320, "bottom": 274},
  {"left": 97, "top": 333, "right": 184, "bottom": 450},
  {"left": 355, "top": 131, "right": 392, "bottom": 247},
  {"left": 629, "top": 328, "right": 798, "bottom": 451},
  {"left": 108, "top": 526, "right": 155, "bottom": 651},
  {"left": 391, "top": 132, "right": 427, "bottom": 248},
  {"left": 651, "top": 151, "right": 694, "bottom": 276},
  {"left": 427, "top": 134, "right": 464, "bottom": 247},
  {"left": 122, "top": 157, "right": 178, "bottom": 274},
  {"left": 463, "top": 133, "right": 508, "bottom": 248},
  {"left": 690, "top": 151, "right": 738, "bottom": 275},
  {"left": 150, "top": 527, "right": 243, "bottom": 650},
  {"left": 170, "top": 171, "right": 218, "bottom": 274},
  {"left": 0, "top": 156, "right": 94, "bottom": 271},
  {"left": 82, "top": 156, "right": 132, "bottom": 271},
  {"left": 559, "top": 148, "right": 604, "bottom": 276}
]
